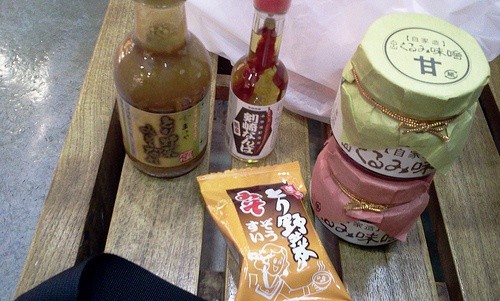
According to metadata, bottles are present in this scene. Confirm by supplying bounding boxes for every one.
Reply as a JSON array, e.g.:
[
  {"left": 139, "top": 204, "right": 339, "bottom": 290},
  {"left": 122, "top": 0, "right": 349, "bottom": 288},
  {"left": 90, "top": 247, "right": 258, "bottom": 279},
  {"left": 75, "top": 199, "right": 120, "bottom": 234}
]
[
  {"left": 113, "top": 0, "right": 213, "bottom": 179},
  {"left": 223, "top": 0, "right": 291, "bottom": 164}
]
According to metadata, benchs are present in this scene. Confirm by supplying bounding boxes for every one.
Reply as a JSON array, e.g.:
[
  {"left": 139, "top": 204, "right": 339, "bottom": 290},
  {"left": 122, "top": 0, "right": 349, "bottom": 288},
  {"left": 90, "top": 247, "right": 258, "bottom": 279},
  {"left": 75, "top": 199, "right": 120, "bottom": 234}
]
[{"left": 13, "top": 0, "right": 500, "bottom": 301}]
